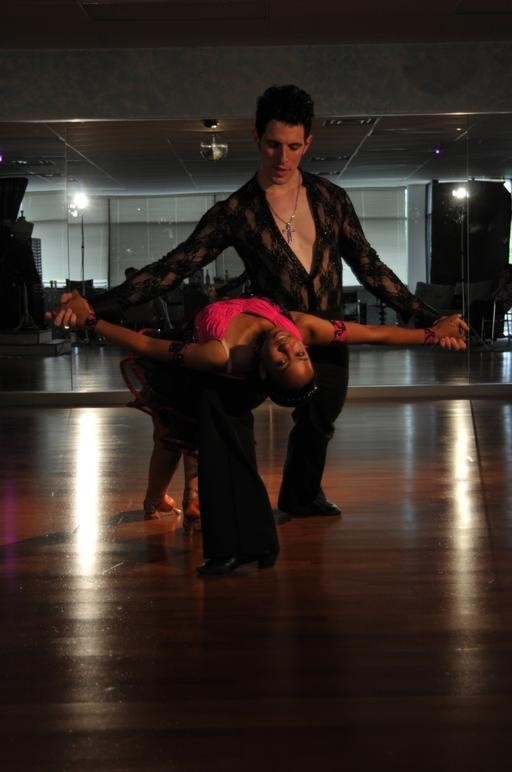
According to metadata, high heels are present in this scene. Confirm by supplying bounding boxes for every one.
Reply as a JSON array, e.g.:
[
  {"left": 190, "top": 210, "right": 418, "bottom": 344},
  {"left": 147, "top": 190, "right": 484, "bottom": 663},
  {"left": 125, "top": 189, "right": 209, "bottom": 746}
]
[
  {"left": 144, "top": 495, "right": 177, "bottom": 520},
  {"left": 182, "top": 516, "right": 201, "bottom": 533},
  {"left": 197, "top": 545, "right": 280, "bottom": 575},
  {"left": 278, "top": 487, "right": 341, "bottom": 514}
]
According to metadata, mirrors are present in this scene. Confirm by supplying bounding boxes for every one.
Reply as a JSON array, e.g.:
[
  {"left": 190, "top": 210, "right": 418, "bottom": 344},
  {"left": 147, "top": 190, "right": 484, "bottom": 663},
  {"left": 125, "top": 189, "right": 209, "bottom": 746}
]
[
  {"left": 5, "top": 121, "right": 258, "bottom": 393},
  {"left": 303, "top": 122, "right": 512, "bottom": 387}
]
